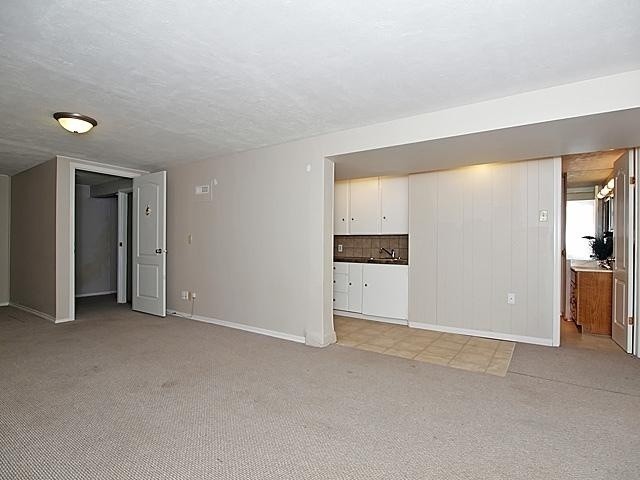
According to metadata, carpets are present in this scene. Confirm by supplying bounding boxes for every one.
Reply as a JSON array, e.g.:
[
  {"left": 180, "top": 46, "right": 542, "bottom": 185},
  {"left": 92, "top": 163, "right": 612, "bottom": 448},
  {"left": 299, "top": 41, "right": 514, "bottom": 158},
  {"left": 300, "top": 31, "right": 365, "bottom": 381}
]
[{"left": 332, "top": 314, "right": 517, "bottom": 377}]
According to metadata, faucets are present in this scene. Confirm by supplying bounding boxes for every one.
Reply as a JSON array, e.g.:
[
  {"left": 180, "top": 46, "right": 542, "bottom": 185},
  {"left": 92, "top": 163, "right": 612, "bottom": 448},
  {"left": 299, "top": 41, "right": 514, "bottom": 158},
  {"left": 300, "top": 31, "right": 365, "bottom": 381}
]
[{"left": 379, "top": 247, "right": 395, "bottom": 258}]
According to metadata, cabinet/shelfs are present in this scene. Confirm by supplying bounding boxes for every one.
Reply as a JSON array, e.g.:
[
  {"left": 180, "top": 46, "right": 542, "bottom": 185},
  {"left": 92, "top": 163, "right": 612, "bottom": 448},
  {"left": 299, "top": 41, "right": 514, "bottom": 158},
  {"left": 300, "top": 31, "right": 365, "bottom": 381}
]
[
  {"left": 334, "top": 177, "right": 380, "bottom": 236},
  {"left": 380, "top": 176, "right": 409, "bottom": 235},
  {"left": 333, "top": 262, "right": 409, "bottom": 320}
]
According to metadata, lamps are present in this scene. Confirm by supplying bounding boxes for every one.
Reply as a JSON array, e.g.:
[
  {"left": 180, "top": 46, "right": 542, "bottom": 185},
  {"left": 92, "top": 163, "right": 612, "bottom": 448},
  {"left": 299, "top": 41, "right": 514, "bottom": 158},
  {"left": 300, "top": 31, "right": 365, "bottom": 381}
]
[{"left": 53, "top": 112, "right": 97, "bottom": 134}]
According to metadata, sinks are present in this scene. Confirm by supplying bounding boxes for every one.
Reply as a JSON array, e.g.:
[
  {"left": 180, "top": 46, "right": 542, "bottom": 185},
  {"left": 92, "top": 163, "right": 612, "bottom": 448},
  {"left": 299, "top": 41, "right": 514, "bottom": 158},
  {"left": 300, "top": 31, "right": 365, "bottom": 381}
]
[{"left": 370, "top": 257, "right": 398, "bottom": 261}]
